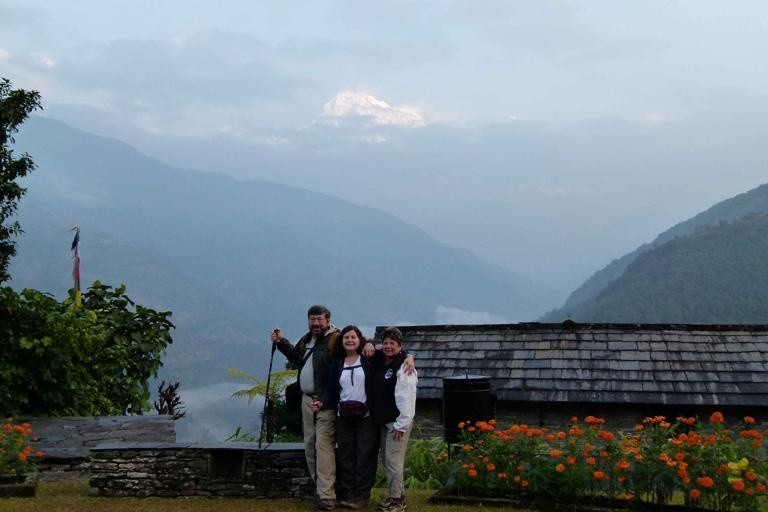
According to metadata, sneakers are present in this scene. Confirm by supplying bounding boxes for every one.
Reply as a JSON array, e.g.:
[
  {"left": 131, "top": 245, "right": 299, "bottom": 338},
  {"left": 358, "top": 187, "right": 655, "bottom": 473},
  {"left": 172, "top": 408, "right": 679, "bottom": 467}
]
[
  {"left": 376, "top": 496, "right": 406, "bottom": 512},
  {"left": 319, "top": 498, "right": 367, "bottom": 512}
]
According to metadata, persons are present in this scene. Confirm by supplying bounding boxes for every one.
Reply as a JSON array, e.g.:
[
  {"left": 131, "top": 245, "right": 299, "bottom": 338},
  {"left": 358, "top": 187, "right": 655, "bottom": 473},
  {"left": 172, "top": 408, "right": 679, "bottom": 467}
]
[
  {"left": 365, "top": 326, "right": 419, "bottom": 512},
  {"left": 270, "top": 304, "right": 376, "bottom": 510},
  {"left": 310, "top": 325, "right": 416, "bottom": 509}
]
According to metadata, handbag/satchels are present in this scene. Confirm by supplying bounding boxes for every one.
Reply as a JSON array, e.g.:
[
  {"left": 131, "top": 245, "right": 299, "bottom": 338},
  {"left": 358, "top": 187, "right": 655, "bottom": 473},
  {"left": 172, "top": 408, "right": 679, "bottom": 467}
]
[
  {"left": 339, "top": 401, "right": 367, "bottom": 420},
  {"left": 286, "top": 385, "right": 304, "bottom": 411}
]
